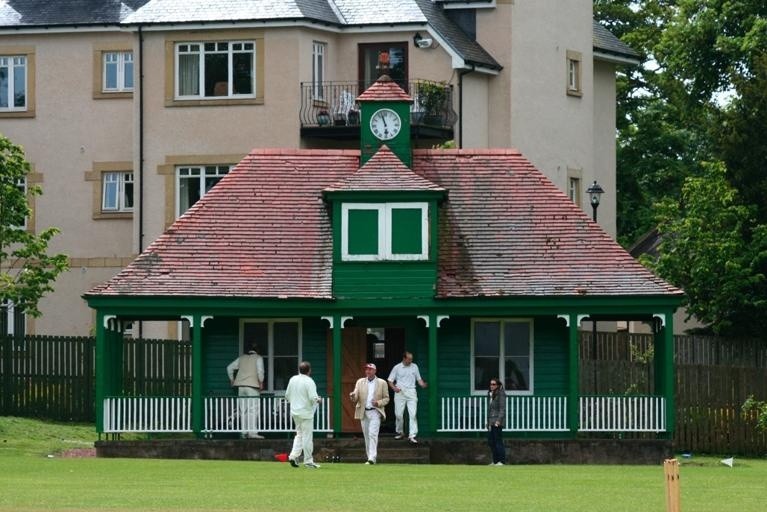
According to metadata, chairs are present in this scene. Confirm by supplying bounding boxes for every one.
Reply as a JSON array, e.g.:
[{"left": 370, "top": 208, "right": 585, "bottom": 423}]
[
  {"left": 213, "top": 81, "right": 232, "bottom": 96},
  {"left": 333, "top": 90, "right": 362, "bottom": 126}
]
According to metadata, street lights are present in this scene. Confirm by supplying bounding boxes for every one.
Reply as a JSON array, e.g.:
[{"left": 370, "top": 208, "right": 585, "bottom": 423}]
[{"left": 584, "top": 179, "right": 608, "bottom": 398}]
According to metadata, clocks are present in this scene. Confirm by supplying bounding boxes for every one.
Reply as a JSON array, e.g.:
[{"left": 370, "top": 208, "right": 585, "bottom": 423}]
[{"left": 368, "top": 108, "right": 401, "bottom": 141}]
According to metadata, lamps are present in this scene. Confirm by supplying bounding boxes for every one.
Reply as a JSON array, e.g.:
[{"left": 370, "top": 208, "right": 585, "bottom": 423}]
[{"left": 416, "top": 39, "right": 439, "bottom": 50}]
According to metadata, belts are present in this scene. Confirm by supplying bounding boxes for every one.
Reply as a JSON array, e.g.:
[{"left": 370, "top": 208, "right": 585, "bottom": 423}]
[{"left": 365, "top": 407, "right": 376, "bottom": 410}]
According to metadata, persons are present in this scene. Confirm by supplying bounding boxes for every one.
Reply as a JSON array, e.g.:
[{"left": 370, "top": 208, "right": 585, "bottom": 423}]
[
  {"left": 284, "top": 361, "right": 322, "bottom": 469},
  {"left": 349, "top": 363, "right": 390, "bottom": 464},
  {"left": 226, "top": 344, "right": 264, "bottom": 440},
  {"left": 488, "top": 378, "right": 505, "bottom": 466},
  {"left": 387, "top": 351, "right": 428, "bottom": 444}
]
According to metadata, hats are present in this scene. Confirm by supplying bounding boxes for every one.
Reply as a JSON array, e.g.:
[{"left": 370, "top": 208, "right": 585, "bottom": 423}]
[{"left": 362, "top": 362, "right": 377, "bottom": 370}]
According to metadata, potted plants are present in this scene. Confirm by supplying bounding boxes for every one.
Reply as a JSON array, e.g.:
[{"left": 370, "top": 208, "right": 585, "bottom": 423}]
[{"left": 416, "top": 78, "right": 449, "bottom": 126}]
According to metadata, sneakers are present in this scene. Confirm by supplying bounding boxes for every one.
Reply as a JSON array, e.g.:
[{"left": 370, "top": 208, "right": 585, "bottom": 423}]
[
  {"left": 304, "top": 463, "right": 321, "bottom": 468},
  {"left": 364, "top": 460, "right": 374, "bottom": 465},
  {"left": 288, "top": 457, "right": 300, "bottom": 468},
  {"left": 488, "top": 461, "right": 504, "bottom": 466},
  {"left": 406, "top": 436, "right": 418, "bottom": 445},
  {"left": 241, "top": 434, "right": 265, "bottom": 440},
  {"left": 394, "top": 434, "right": 403, "bottom": 440}
]
